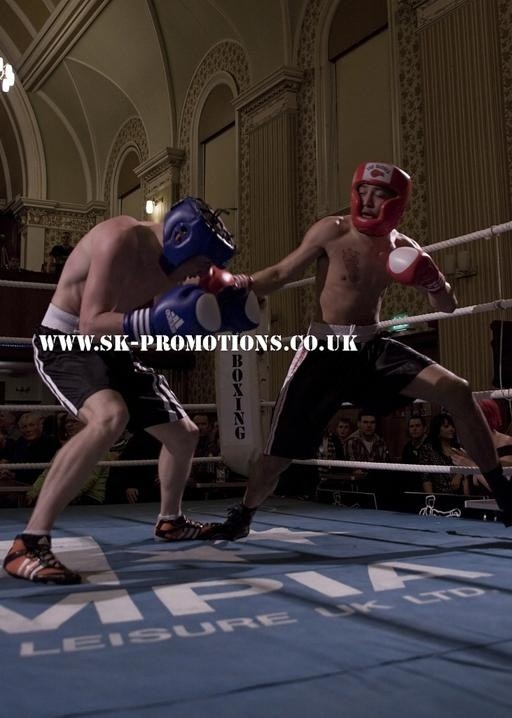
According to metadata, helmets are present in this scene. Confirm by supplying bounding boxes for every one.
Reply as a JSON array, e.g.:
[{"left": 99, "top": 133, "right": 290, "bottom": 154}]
[
  {"left": 160, "top": 194, "right": 237, "bottom": 274},
  {"left": 349, "top": 160, "right": 414, "bottom": 239}
]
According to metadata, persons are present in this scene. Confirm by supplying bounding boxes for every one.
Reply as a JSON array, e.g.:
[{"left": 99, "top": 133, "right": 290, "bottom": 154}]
[
  {"left": 46, "top": 229, "right": 75, "bottom": 276},
  {"left": 1, "top": 396, "right": 511, "bottom": 513},
  {"left": 3, "top": 190, "right": 270, "bottom": 587},
  {"left": 180, "top": 157, "right": 510, "bottom": 543}
]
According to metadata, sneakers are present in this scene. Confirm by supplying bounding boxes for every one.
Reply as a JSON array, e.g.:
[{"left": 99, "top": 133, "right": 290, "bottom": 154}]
[
  {"left": 209, "top": 502, "right": 258, "bottom": 542},
  {"left": 499, "top": 477, "right": 512, "bottom": 528},
  {"left": 1, "top": 531, "right": 86, "bottom": 585},
  {"left": 153, "top": 512, "right": 214, "bottom": 543}
]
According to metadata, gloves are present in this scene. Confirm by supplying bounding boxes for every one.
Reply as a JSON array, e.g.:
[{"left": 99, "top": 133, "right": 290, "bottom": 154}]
[
  {"left": 382, "top": 246, "right": 447, "bottom": 293},
  {"left": 177, "top": 264, "right": 254, "bottom": 293},
  {"left": 119, "top": 284, "right": 223, "bottom": 351},
  {"left": 217, "top": 287, "right": 263, "bottom": 336}
]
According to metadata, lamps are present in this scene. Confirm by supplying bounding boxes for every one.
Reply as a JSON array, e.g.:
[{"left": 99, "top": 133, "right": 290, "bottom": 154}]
[
  {"left": 145, "top": 199, "right": 158, "bottom": 215},
  {"left": 0, "top": 56, "right": 17, "bottom": 93}
]
